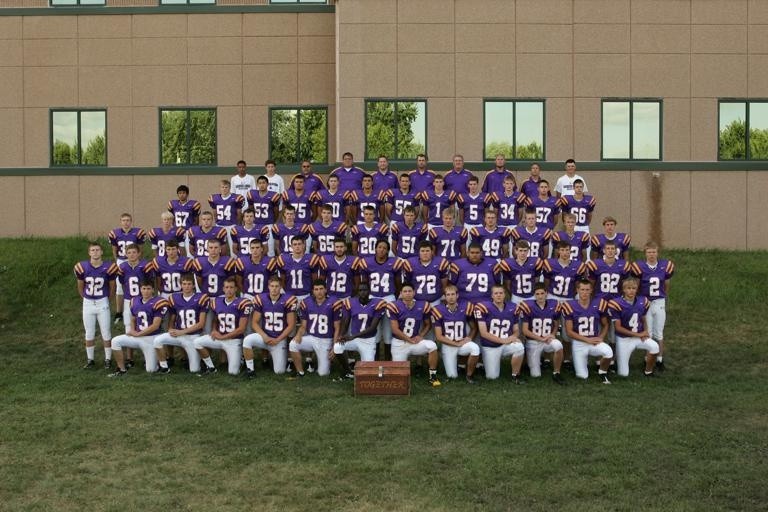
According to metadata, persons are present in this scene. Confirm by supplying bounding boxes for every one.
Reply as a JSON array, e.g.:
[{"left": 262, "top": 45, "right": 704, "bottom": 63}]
[
  {"left": 230, "top": 152, "right": 589, "bottom": 196},
  {"left": 74, "top": 177, "right": 672, "bottom": 387}
]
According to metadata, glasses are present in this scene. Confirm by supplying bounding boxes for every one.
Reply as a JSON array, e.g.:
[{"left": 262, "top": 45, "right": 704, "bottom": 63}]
[{"left": 304, "top": 166, "right": 309, "bottom": 168}]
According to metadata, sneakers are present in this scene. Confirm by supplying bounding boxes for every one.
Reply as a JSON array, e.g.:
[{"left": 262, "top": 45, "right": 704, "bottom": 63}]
[
  {"left": 157, "top": 356, "right": 218, "bottom": 377},
  {"left": 563, "top": 360, "right": 574, "bottom": 377},
  {"left": 83, "top": 357, "right": 146, "bottom": 378},
  {"left": 596, "top": 362, "right": 616, "bottom": 373},
  {"left": 512, "top": 374, "right": 529, "bottom": 385},
  {"left": 287, "top": 357, "right": 315, "bottom": 382},
  {"left": 552, "top": 372, "right": 567, "bottom": 385},
  {"left": 475, "top": 363, "right": 485, "bottom": 375},
  {"left": 540, "top": 356, "right": 550, "bottom": 369},
  {"left": 642, "top": 354, "right": 665, "bottom": 379},
  {"left": 598, "top": 373, "right": 612, "bottom": 385},
  {"left": 218, "top": 354, "right": 273, "bottom": 379},
  {"left": 344, "top": 358, "right": 355, "bottom": 380},
  {"left": 415, "top": 360, "right": 478, "bottom": 387}
]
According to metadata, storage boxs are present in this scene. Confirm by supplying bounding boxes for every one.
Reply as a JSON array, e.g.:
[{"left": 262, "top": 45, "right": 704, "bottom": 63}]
[{"left": 352, "top": 359, "right": 413, "bottom": 397}]
